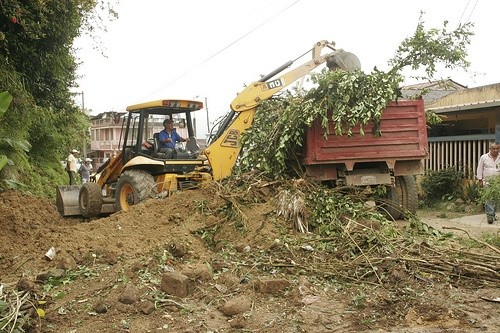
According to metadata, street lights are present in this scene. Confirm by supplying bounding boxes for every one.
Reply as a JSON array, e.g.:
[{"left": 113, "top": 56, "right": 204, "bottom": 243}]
[{"left": 193, "top": 96, "right": 210, "bottom": 133}]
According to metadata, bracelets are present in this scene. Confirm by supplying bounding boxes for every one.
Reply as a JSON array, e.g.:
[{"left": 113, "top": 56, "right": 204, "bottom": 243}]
[{"left": 479, "top": 180, "right": 482, "bottom": 182}]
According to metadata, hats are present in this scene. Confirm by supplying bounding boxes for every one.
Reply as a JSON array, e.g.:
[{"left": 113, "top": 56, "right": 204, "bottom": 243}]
[
  {"left": 72, "top": 149, "right": 79, "bottom": 153},
  {"left": 163, "top": 119, "right": 174, "bottom": 125},
  {"left": 85, "top": 157, "right": 93, "bottom": 162}
]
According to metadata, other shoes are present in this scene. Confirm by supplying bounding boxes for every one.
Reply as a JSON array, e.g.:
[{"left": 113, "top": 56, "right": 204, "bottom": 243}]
[{"left": 487, "top": 217, "right": 493, "bottom": 224}]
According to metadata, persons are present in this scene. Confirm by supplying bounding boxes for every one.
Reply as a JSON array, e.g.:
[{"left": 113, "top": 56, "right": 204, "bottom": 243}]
[
  {"left": 476, "top": 142, "right": 500, "bottom": 224},
  {"left": 157, "top": 119, "right": 190, "bottom": 160},
  {"left": 78, "top": 158, "right": 93, "bottom": 183},
  {"left": 66, "top": 149, "right": 80, "bottom": 185}
]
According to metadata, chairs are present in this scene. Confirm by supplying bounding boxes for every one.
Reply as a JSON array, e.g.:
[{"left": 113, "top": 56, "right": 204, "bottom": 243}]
[{"left": 153, "top": 134, "right": 166, "bottom": 158}]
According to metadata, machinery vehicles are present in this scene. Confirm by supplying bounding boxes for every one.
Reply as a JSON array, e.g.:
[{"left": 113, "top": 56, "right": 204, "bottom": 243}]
[{"left": 56, "top": 40, "right": 361, "bottom": 222}]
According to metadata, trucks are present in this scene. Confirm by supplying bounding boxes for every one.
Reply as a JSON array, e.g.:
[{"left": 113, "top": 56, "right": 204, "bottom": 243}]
[{"left": 287, "top": 96, "right": 429, "bottom": 220}]
